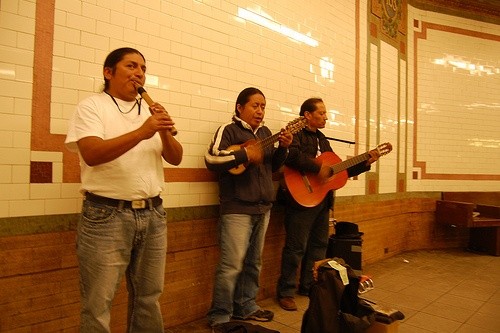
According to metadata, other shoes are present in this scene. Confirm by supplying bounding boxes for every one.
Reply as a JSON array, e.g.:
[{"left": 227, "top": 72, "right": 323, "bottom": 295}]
[{"left": 279, "top": 297, "right": 298, "bottom": 311}]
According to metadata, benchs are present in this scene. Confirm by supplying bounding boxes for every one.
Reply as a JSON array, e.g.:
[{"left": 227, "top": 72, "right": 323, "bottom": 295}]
[{"left": 436, "top": 192, "right": 500, "bottom": 256}]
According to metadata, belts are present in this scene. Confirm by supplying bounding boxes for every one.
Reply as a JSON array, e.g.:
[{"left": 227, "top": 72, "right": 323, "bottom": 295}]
[{"left": 86, "top": 192, "right": 163, "bottom": 211}]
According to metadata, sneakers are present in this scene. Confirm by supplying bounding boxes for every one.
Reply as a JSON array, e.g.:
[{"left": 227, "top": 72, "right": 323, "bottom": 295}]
[{"left": 241, "top": 309, "right": 275, "bottom": 322}]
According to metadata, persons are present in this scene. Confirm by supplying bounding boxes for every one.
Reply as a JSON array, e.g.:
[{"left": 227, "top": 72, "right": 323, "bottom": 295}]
[
  {"left": 64, "top": 48, "right": 182, "bottom": 333},
  {"left": 204, "top": 87, "right": 293, "bottom": 333},
  {"left": 277, "top": 98, "right": 380, "bottom": 311}
]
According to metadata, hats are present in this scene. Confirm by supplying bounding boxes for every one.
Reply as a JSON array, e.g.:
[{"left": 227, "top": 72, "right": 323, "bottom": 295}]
[{"left": 331, "top": 220, "right": 365, "bottom": 239}]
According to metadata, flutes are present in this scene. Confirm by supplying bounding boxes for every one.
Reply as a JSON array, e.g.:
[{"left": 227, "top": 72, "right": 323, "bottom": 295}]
[{"left": 132, "top": 81, "right": 177, "bottom": 136}]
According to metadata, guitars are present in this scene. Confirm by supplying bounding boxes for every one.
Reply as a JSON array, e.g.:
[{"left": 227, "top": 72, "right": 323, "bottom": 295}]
[
  {"left": 224, "top": 116, "right": 308, "bottom": 175},
  {"left": 279, "top": 142, "right": 393, "bottom": 209}
]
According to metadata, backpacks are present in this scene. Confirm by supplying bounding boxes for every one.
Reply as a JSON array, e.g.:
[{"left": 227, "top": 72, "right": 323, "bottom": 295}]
[{"left": 301, "top": 256, "right": 376, "bottom": 333}]
